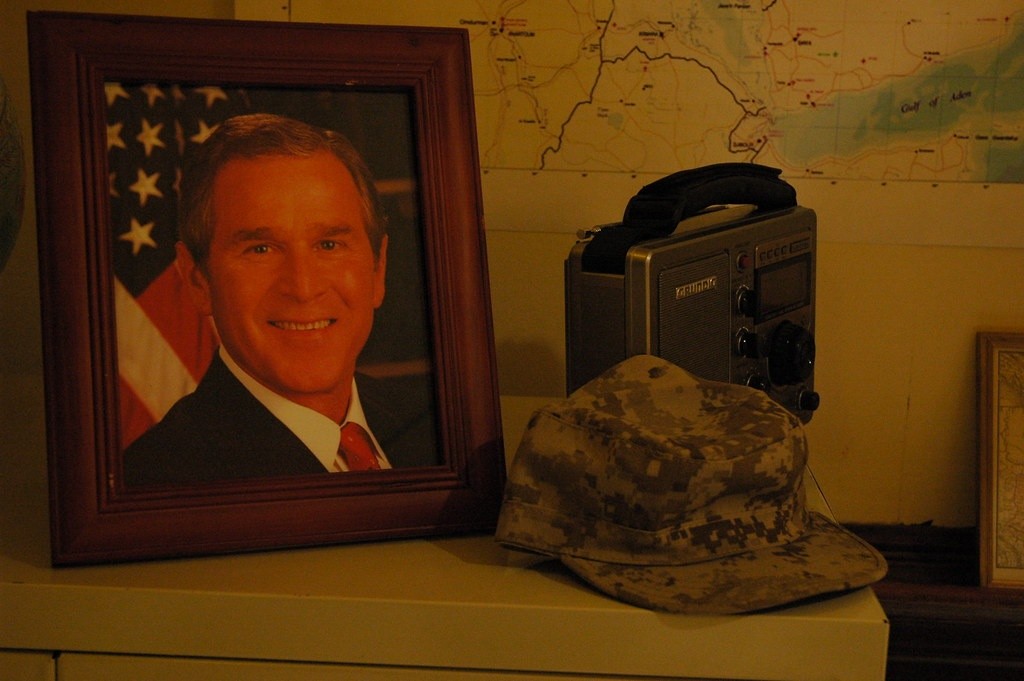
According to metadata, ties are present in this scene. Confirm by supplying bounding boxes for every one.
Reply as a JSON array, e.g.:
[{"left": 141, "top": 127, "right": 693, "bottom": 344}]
[{"left": 338, "top": 424, "right": 380, "bottom": 471}]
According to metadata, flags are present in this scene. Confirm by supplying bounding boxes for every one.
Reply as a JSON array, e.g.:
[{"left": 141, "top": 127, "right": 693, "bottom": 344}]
[{"left": 89, "top": 83, "right": 228, "bottom": 446}]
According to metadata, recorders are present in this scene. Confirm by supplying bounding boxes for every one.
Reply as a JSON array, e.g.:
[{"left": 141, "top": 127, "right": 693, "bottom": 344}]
[{"left": 563, "top": 163, "right": 820, "bottom": 425}]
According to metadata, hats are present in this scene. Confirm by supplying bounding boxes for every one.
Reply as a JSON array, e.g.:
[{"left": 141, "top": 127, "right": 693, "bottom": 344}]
[{"left": 492, "top": 352, "right": 890, "bottom": 615}]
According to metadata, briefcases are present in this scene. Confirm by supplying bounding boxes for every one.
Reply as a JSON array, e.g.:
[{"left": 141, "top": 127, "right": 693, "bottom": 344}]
[{"left": 564, "top": 162, "right": 818, "bottom": 422}]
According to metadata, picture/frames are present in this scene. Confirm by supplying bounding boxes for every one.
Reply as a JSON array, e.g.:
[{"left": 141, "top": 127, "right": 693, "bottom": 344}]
[
  {"left": 24, "top": 9, "right": 506, "bottom": 570},
  {"left": 977, "top": 331, "right": 1024, "bottom": 590}
]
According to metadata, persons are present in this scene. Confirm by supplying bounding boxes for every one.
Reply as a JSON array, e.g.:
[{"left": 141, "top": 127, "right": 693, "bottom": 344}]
[{"left": 110, "top": 113, "right": 449, "bottom": 482}]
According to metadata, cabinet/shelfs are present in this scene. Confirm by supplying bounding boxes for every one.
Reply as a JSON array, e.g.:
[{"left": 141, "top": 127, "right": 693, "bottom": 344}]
[{"left": 0, "top": 394, "right": 893, "bottom": 681}]
